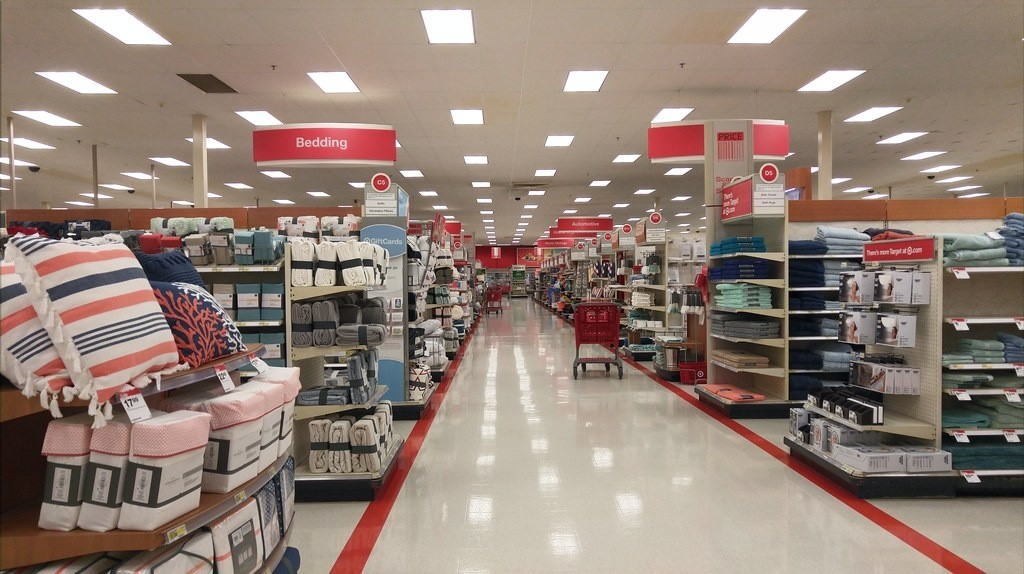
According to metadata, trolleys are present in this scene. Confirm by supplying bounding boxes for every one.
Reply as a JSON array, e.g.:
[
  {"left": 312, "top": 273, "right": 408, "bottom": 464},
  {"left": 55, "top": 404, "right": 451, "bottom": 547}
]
[
  {"left": 486, "top": 286, "right": 503, "bottom": 314},
  {"left": 570, "top": 300, "right": 623, "bottom": 379}
]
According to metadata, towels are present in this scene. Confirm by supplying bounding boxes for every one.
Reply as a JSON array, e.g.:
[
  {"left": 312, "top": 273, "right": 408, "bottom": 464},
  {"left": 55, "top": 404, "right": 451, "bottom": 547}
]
[{"left": 925, "top": 213, "right": 1024, "bottom": 471}]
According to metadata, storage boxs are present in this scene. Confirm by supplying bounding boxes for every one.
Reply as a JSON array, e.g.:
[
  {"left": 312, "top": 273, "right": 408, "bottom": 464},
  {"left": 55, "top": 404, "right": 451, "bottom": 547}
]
[
  {"left": 789, "top": 407, "right": 953, "bottom": 472},
  {"left": 837, "top": 310, "right": 917, "bottom": 348},
  {"left": 838, "top": 270, "right": 932, "bottom": 307},
  {"left": 849, "top": 360, "right": 921, "bottom": 396}
]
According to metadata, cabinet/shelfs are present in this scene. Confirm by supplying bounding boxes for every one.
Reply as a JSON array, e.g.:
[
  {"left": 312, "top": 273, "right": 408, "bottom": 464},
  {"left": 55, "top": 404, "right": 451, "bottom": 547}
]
[
  {"left": 694, "top": 197, "right": 863, "bottom": 419},
  {"left": 0, "top": 207, "right": 488, "bottom": 574},
  {"left": 609, "top": 229, "right": 706, "bottom": 362},
  {"left": 783, "top": 235, "right": 1024, "bottom": 498},
  {"left": 539, "top": 249, "right": 607, "bottom": 315}
]
